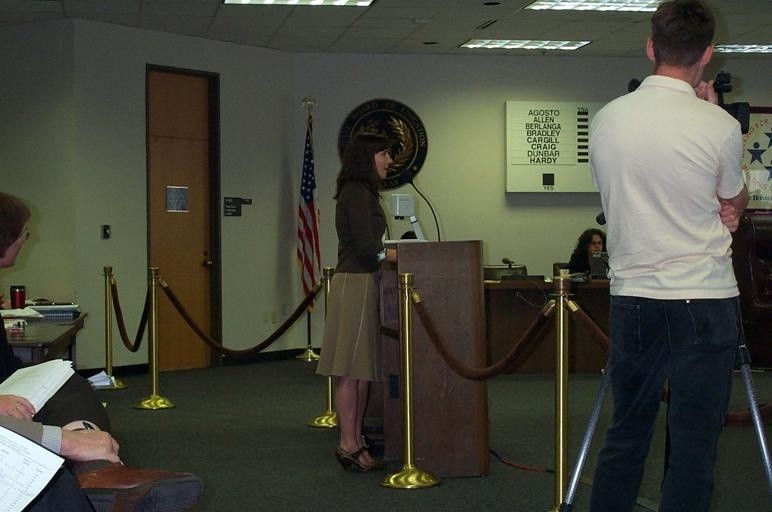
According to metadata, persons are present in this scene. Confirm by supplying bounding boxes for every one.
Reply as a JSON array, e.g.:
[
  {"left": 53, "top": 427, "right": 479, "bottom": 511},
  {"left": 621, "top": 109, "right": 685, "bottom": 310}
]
[
  {"left": 1, "top": 356, "right": 205, "bottom": 511},
  {"left": 587, "top": 0, "right": 750, "bottom": 509},
  {"left": 314, "top": 133, "right": 395, "bottom": 474},
  {"left": 567, "top": 229, "right": 608, "bottom": 278},
  {"left": 0, "top": 194, "right": 33, "bottom": 384}
]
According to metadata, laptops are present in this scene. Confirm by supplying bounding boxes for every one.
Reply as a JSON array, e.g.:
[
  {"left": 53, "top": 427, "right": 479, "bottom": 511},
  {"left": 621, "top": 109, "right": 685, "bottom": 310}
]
[{"left": 588, "top": 251, "right": 611, "bottom": 279}]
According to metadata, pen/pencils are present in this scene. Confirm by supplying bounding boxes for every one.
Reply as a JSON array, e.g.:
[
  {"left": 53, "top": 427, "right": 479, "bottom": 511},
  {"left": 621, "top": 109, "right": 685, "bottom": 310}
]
[{"left": 83, "top": 422, "right": 126, "bottom": 466}]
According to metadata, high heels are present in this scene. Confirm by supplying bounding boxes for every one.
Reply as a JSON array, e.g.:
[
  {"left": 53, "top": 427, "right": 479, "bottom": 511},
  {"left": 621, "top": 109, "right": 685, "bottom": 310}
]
[
  {"left": 359, "top": 445, "right": 388, "bottom": 469},
  {"left": 335, "top": 446, "right": 382, "bottom": 474}
]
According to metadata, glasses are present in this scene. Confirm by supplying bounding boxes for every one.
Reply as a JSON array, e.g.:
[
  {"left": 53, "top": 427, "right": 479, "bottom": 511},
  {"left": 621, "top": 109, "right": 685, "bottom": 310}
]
[{"left": 18, "top": 227, "right": 32, "bottom": 241}]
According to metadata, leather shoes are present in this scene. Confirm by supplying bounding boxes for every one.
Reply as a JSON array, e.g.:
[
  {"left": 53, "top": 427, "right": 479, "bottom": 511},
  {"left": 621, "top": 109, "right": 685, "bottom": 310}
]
[{"left": 69, "top": 460, "right": 206, "bottom": 512}]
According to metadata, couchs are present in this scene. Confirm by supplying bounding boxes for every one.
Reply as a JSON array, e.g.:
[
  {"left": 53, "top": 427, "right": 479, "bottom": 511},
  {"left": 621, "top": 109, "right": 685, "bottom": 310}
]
[{"left": 732, "top": 214, "right": 772, "bottom": 371}]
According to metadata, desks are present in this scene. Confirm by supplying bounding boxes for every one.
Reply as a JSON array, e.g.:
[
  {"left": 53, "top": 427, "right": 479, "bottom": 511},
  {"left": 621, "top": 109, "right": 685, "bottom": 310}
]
[
  {"left": 0, "top": 297, "right": 88, "bottom": 379},
  {"left": 485, "top": 274, "right": 611, "bottom": 375}
]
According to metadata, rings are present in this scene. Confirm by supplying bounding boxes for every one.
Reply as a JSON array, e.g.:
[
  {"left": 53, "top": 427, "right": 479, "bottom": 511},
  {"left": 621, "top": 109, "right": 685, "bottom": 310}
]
[{"left": 15, "top": 401, "right": 23, "bottom": 408}]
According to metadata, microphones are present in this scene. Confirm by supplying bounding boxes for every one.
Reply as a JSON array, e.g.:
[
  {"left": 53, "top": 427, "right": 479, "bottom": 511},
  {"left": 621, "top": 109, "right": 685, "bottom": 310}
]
[
  {"left": 501, "top": 256, "right": 512, "bottom": 268},
  {"left": 401, "top": 171, "right": 443, "bottom": 242}
]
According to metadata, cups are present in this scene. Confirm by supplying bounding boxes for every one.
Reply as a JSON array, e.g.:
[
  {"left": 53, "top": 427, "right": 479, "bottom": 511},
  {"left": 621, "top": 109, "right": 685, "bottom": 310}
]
[{"left": 10, "top": 285, "right": 25, "bottom": 310}]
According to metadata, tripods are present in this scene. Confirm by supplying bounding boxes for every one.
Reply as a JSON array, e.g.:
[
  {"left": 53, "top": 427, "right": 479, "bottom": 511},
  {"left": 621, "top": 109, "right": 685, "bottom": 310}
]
[{"left": 557, "top": 341, "right": 771, "bottom": 512}]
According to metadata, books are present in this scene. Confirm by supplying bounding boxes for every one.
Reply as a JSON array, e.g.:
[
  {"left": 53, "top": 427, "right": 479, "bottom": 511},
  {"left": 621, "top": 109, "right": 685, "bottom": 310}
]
[{"left": 40, "top": 311, "right": 81, "bottom": 317}]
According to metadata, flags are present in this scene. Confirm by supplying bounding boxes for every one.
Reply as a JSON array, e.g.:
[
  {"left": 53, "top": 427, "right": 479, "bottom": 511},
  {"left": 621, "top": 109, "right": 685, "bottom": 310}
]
[{"left": 295, "top": 117, "right": 323, "bottom": 313}]
